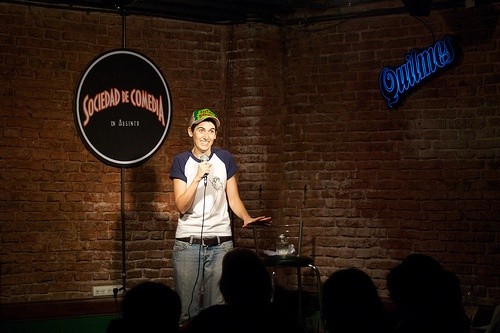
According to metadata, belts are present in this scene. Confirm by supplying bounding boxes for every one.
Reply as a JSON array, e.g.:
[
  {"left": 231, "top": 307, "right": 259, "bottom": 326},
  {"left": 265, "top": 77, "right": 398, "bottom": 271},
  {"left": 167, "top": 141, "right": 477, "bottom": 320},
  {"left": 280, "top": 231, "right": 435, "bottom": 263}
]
[{"left": 174, "top": 236, "right": 233, "bottom": 245}]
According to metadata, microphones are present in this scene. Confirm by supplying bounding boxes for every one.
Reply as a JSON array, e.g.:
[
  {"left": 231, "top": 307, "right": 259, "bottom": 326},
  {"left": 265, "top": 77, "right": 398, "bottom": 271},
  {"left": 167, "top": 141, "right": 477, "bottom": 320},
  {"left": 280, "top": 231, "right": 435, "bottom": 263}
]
[{"left": 200, "top": 154, "right": 210, "bottom": 186}]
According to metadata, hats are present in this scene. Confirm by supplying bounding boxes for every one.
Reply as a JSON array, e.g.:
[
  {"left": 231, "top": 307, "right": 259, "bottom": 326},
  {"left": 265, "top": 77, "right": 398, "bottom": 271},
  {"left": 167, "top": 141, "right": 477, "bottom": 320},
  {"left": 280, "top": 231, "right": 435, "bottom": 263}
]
[{"left": 188, "top": 108, "right": 220, "bottom": 129}]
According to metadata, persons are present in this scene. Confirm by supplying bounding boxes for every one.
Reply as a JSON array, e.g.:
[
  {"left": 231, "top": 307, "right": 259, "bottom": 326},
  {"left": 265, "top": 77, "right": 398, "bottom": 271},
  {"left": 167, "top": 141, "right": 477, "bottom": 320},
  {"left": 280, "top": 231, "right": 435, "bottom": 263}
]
[
  {"left": 182, "top": 248, "right": 306, "bottom": 332},
  {"left": 105, "top": 281, "right": 182, "bottom": 333},
  {"left": 169, "top": 107, "right": 273, "bottom": 326},
  {"left": 385, "top": 253, "right": 473, "bottom": 333},
  {"left": 320, "top": 267, "right": 396, "bottom": 332}
]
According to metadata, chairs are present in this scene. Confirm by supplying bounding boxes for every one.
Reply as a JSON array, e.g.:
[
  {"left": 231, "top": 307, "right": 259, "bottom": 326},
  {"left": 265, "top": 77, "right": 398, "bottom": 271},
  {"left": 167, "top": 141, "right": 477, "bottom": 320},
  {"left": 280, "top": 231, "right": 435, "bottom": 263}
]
[{"left": 250, "top": 218, "right": 324, "bottom": 314}]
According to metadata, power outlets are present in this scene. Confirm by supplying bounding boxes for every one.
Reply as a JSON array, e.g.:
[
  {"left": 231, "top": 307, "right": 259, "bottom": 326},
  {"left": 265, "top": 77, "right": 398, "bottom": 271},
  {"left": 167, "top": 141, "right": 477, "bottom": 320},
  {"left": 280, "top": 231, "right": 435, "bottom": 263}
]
[{"left": 92, "top": 284, "right": 124, "bottom": 295}]
